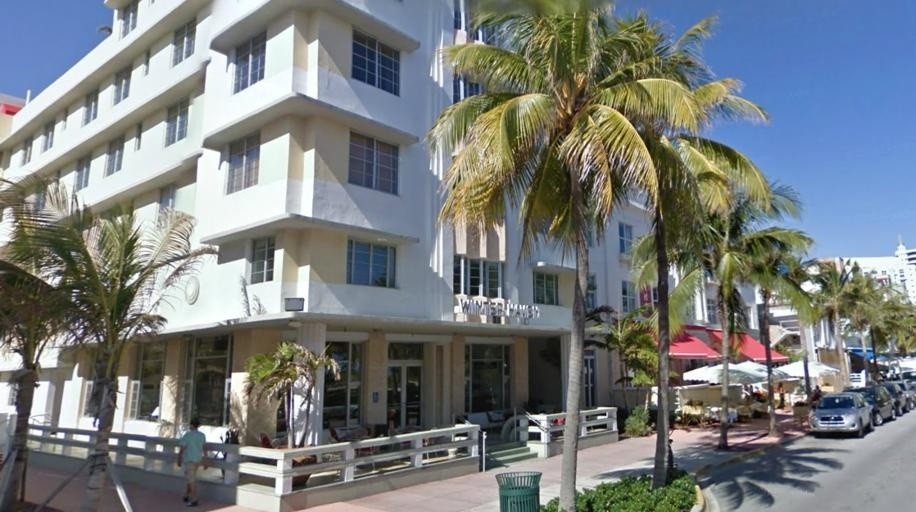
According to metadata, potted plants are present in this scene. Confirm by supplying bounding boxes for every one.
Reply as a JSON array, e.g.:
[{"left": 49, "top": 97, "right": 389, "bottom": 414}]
[
  {"left": 791, "top": 400, "right": 809, "bottom": 417},
  {"left": 241, "top": 331, "right": 341, "bottom": 489}
]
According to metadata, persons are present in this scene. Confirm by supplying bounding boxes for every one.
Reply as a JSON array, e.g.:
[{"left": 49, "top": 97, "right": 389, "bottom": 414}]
[
  {"left": 175, "top": 417, "right": 209, "bottom": 507},
  {"left": 812, "top": 386, "right": 822, "bottom": 403},
  {"left": 776, "top": 384, "right": 785, "bottom": 408}
]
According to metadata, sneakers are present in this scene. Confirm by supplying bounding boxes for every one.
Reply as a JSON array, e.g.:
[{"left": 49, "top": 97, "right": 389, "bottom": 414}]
[{"left": 183, "top": 494, "right": 198, "bottom": 507}]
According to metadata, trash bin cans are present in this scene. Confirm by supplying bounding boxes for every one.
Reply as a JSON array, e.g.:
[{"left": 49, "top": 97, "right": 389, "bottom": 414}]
[{"left": 495, "top": 472, "right": 542, "bottom": 512}]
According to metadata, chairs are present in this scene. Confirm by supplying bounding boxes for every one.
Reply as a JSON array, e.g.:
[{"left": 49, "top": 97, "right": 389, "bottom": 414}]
[
  {"left": 482, "top": 400, "right": 596, "bottom": 440},
  {"left": 258, "top": 420, "right": 475, "bottom": 481}
]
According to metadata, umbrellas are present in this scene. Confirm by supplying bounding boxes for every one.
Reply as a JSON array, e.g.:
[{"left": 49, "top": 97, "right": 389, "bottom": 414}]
[{"left": 680, "top": 359, "right": 840, "bottom": 386}]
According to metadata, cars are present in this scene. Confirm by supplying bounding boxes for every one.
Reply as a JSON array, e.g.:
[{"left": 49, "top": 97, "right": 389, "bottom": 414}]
[
  {"left": 322, "top": 380, "right": 492, "bottom": 424},
  {"left": 843, "top": 367, "right": 916, "bottom": 437},
  {"left": 807, "top": 393, "right": 873, "bottom": 438}
]
